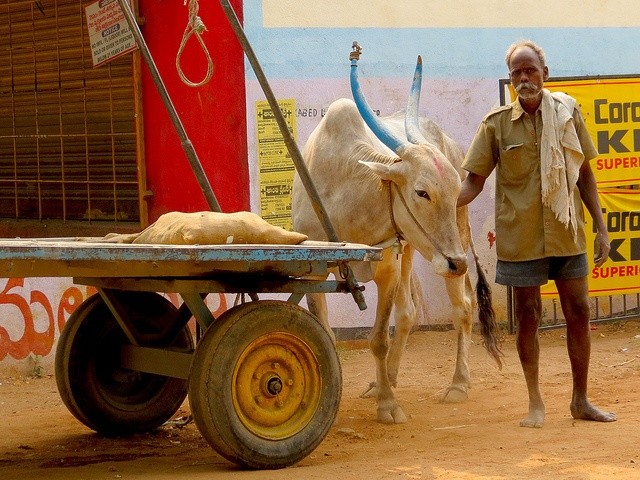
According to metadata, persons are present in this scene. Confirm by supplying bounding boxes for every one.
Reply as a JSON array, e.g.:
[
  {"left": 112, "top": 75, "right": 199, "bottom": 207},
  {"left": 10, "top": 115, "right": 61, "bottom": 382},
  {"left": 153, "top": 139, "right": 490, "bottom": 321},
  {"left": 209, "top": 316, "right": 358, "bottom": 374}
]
[{"left": 457, "top": 39, "right": 618, "bottom": 430}]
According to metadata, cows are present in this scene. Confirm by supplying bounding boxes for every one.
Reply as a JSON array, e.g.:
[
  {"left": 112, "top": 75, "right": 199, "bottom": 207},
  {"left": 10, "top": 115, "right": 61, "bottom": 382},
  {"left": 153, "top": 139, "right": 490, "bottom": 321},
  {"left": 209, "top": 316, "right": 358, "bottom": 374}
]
[{"left": 292, "top": 55, "right": 505, "bottom": 425}]
